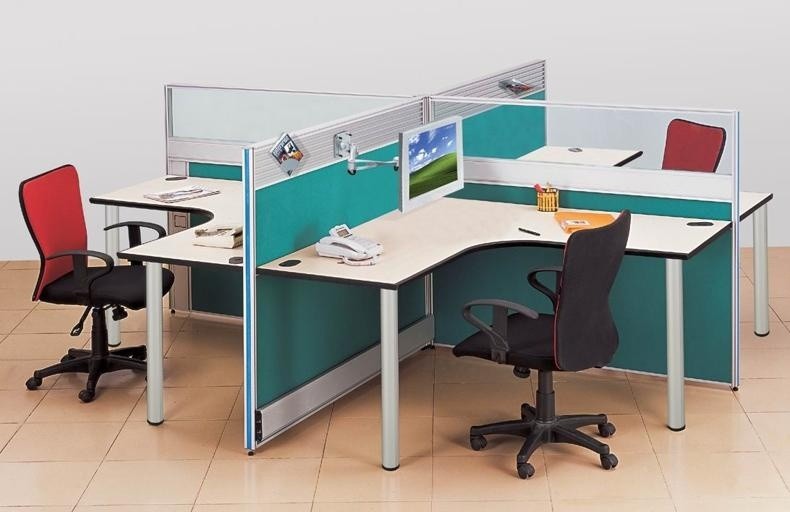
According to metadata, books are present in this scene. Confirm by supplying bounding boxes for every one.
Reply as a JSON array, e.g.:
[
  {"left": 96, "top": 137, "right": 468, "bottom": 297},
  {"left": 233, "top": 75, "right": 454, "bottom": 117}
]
[
  {"left": 267, "top": 131, "right": 304, "bottom": 177},
  {"left": 144, "top": 183, "right": 220, "bottom": 203}
]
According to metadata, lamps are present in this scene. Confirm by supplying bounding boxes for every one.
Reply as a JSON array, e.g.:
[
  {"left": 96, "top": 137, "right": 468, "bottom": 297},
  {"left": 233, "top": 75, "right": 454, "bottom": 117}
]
[{"left": 333, "top": 130, "right": 400, "bottom": 176}]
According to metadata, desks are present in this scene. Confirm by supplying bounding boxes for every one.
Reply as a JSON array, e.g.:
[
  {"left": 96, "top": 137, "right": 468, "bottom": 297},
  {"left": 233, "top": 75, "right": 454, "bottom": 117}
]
[
  {"left": 511, "top": 146, "right": 775, "bottom": 336},
  {"left": 88, "top": 171, "right": 244, "bottom": 425},
  {"left": 256, "top": 188, "right": 733, "bottom": 470}
]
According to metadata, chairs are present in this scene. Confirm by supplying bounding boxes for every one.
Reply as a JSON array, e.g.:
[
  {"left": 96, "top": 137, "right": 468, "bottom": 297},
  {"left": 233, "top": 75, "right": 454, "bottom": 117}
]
[
  {"left": 661, "top": 118, "right": 728, "bottom": 174},
  {"left": 18, "top": 164, "right": 174, "bottom": 403},
  {"left": 450, "top": 207, "right": 635, "bottom": 480}
]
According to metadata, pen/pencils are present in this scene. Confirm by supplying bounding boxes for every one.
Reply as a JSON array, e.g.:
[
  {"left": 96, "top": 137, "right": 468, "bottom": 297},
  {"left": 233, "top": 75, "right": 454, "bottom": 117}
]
[
  {"left": 535, "top": 182, "right": 552, "bottom": 192},
  {"left": 165, "top": 177, "right": 187, "bottom": 180},
  {"left": 518, "top": 228, "right": 540, "bottom": 236}
]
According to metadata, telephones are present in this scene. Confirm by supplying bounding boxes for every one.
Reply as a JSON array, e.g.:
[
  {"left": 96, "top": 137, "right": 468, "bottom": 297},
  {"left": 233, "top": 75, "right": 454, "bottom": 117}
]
[
  {"left": 315, "top": 224, "right": 384, "bottom": 260},
  {"left": 192, "top": 226, "right": 242, "bottom": 248}
]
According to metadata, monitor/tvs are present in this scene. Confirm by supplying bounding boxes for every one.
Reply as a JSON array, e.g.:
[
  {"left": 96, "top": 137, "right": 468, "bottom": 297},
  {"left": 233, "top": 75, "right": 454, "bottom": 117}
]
[{"left": 334, "top": 116, "right": 464, "bottom": 214}]
